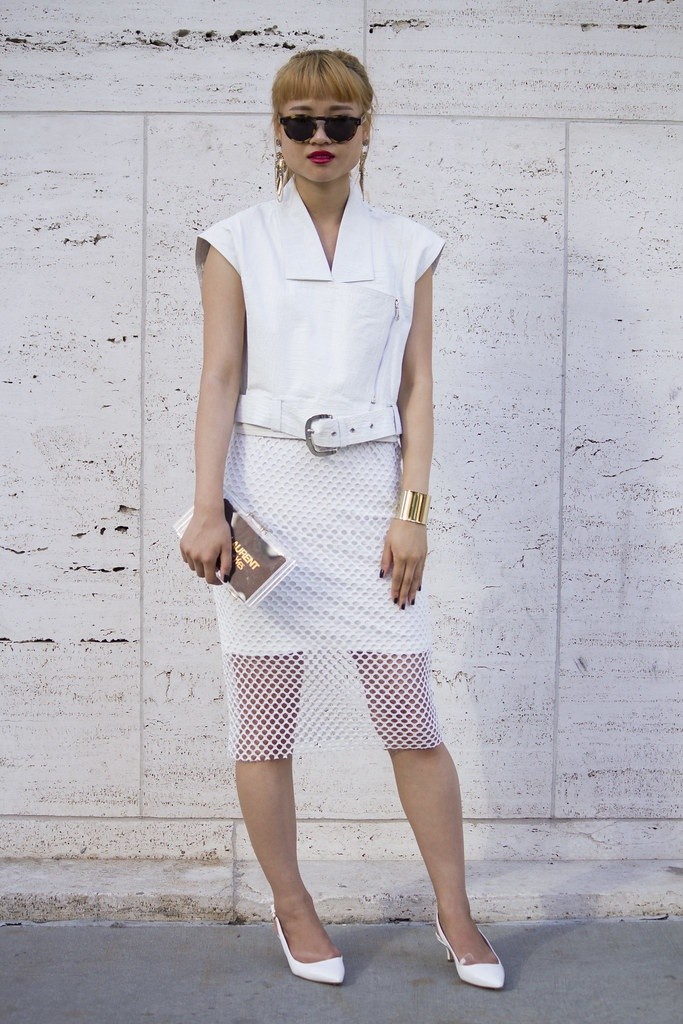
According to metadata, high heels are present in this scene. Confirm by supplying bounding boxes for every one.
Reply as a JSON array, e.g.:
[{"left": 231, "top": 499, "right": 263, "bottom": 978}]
[
  {"left": 433, "top": 910, "right": 506, "bottom": 989},
  {"left": 269, "top": 904, "right": 345, "bottom": 985}
]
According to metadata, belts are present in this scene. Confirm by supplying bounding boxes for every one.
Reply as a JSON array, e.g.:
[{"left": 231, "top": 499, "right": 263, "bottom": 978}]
[{"left": 235, "top": 394, "right": 402, "bottom": 456}]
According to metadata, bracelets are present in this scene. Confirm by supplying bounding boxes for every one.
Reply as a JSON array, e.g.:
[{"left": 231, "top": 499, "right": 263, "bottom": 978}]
[{"left": 394, "top": 490, "right": 432, "bottom": 525}]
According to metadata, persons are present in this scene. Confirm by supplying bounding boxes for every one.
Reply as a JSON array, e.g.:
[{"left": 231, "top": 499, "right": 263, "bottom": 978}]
[{"left": 180, "top": 49, "right": 506, "bottom": 988}]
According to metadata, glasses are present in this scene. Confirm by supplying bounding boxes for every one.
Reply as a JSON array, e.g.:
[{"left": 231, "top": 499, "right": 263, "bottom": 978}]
[{"left": 278, "top": 108, "right": 366, "bottom": 146}]
